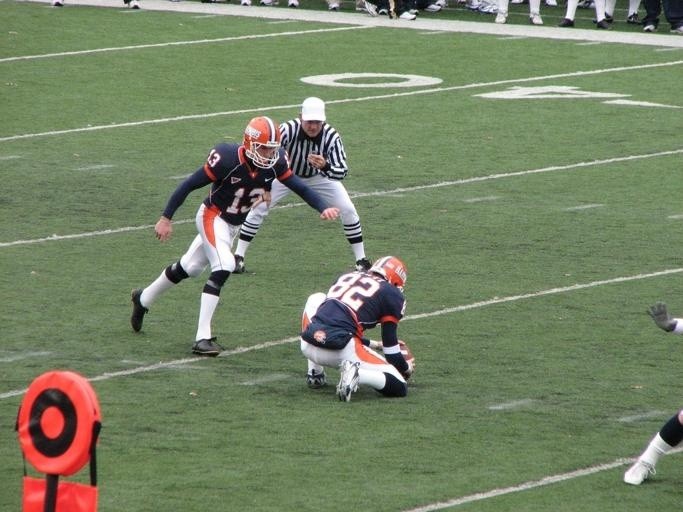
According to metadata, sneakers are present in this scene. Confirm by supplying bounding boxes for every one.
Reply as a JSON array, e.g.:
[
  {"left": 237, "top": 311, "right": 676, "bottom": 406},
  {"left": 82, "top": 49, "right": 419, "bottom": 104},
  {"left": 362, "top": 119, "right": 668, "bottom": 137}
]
[
  {"left": 624, "top": 456, "right": 656, "bottom": 485},
  {"left": 305, "top": 368, "right": 327, "bottom": 389},
  {"left": 335, "top": 359, "right": 361, "bottom": 403},
  {"left": 52, "top": 0, "right": 65, "bottom": 6},
  {"left": 128, "top": 0, "right": 141, "bottom": 9},
  {"left": 243, "top": 0, "right": 447, "bottom": 20},
  {"left": 130, "top": 288, "right": 149, "bottom": 332},
  {"left": 466, "top": 1, "right": 674, "bottom": 33},
  {"left": 191, "top": 338, "right": 220, "bottom": 356},
  {"left": 231, "top": 254, "right": 246, "bottom": 274},
  {"left": 355, "top": 257, "right": 372, "bottom": 272}
]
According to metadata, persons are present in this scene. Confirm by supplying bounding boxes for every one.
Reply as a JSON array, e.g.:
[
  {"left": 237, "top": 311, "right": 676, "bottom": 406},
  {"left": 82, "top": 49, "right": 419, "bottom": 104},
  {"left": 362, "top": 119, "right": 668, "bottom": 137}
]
[
  {"left": 232, "top": 94, "right": 371, "bottom": 272},
  {"left": 49, "top": 0, "right": 142, "bottom": 10},
  {"left": 170, "top": 0, "right": 682, "bottom": 36},
  {"left": 298, "top": 252, "right": 415, "bottom": 404},
  {"left": 622, "top": 298, "right": 683, "bottom": 486},
  {"left": 127, "top": 113, "right": 341, "bottom": 357}
]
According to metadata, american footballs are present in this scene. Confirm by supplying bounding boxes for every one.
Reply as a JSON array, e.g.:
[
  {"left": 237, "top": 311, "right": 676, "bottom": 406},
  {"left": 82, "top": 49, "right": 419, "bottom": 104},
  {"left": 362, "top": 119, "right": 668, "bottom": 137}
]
[{"left": 397, "top": 343, "right": 416, "bottom": 370}]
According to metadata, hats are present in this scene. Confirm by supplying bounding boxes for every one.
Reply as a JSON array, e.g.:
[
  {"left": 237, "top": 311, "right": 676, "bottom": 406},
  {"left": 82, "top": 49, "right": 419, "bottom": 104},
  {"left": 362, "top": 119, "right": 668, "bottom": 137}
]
[{"left": 301, "top": 97, "right": 326, "bottom": 122}]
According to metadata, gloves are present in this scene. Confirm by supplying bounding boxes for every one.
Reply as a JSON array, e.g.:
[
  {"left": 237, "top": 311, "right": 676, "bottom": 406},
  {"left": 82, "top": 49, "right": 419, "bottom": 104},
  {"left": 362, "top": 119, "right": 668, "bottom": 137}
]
[{"left": 645, "top": 302, "right": 677, "bottom": 332}]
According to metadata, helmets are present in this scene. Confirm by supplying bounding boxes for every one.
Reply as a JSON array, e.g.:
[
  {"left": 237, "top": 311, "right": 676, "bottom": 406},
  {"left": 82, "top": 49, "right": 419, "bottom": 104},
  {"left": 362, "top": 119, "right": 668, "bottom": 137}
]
[
  {"left": 368, "top": 255, "right": 407, "bottom": 293},
  {"left": 243, "top": 116, "right": 282, "bottom": 170}
]
